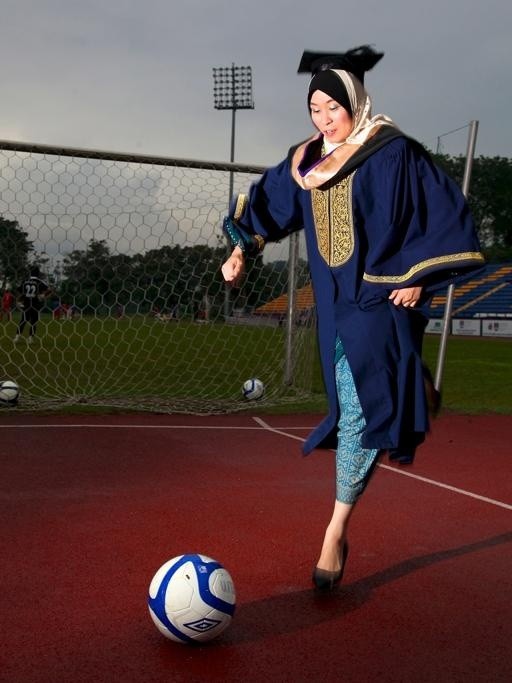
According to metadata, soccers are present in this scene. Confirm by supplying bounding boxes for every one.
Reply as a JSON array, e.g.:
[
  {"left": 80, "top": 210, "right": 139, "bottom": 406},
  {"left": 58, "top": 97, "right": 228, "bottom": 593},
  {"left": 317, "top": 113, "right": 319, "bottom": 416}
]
[
  {"left": 242, "top": 379, "right": 264, "bottom": 399},
  {"left": 149, "top": 554, "right": 236, "bottom": 643},
  {"left": 0, "top": 380, "right": 22, "bottom": 407}
]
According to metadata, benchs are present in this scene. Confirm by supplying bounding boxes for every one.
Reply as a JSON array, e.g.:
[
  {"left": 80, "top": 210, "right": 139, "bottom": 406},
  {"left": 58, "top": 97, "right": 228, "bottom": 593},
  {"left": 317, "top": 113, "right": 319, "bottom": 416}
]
[{"left": 256, "top": 263, "right": 512, "bottom": 321}]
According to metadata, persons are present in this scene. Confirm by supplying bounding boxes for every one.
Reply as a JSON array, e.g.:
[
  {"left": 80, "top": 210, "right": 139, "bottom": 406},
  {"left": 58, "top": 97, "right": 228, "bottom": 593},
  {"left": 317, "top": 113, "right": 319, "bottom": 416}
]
[
  {"left": 14, "top": 263, "right": 55, "bottom": 338},
  {"left": 1, "top": 288, "right": 13, "bottom": 322},
  {"left": 149, "top": 304, "right": 209, "bottom": 321},
  {"left": 215, "top": 68, "right": 487, "bottom": 585},
  {"left": 51, "top": 300, "right": 78, "bottom": 320}
]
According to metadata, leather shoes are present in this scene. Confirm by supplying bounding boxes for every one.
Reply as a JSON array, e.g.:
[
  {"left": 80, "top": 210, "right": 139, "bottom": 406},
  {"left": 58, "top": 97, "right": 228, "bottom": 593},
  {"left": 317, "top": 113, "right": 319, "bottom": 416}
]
[{"left": 310, "top": 537, "right": 351, "bottom": 592}]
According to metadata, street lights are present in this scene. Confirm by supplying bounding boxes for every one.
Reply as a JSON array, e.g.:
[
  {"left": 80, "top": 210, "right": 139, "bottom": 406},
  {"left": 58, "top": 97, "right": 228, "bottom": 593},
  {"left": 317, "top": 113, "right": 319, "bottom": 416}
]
[{"left": 213, "top": 62, "right": 255, "bottom": 320}]
